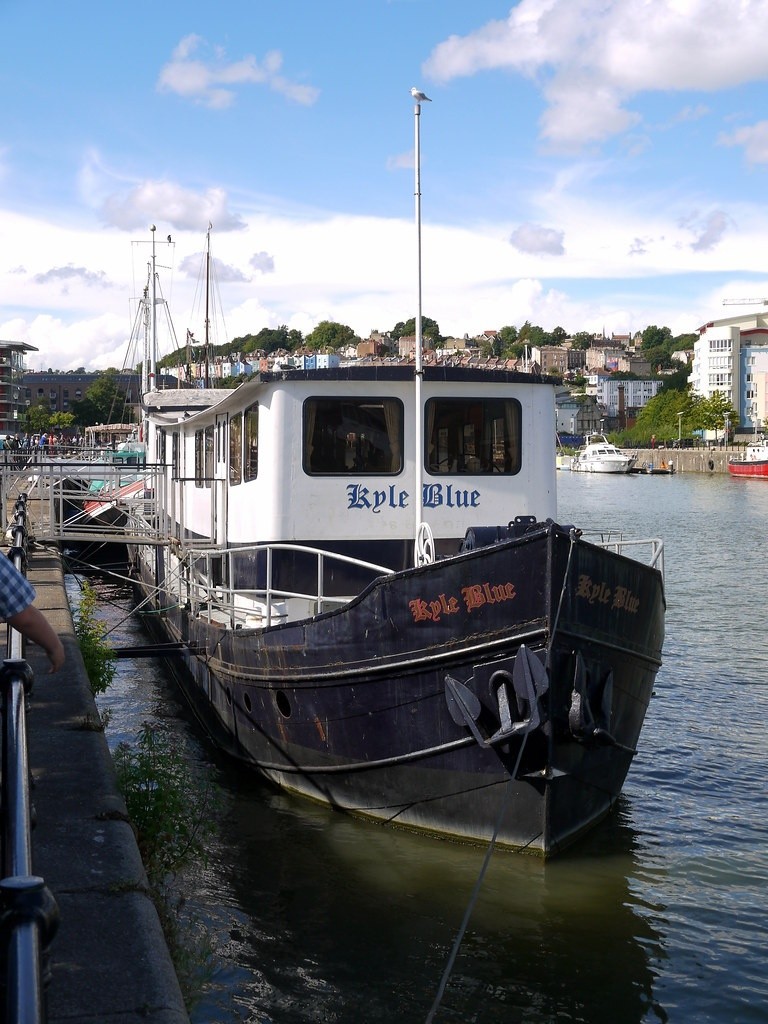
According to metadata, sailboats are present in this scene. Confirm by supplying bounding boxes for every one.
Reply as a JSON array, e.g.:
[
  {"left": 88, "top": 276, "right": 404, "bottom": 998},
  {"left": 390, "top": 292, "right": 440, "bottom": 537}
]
[{"left": 75, "top": 222, "right": 241, "bottom": 543}]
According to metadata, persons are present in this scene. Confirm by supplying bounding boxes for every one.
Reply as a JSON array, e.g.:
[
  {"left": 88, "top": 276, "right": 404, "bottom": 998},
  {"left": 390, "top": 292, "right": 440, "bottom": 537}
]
[
  {"left": 0, "top": 550, "right": 66, "bottom": 674},
  {"left": 0, "top": 433, "right": 99, "bottom": 450}
]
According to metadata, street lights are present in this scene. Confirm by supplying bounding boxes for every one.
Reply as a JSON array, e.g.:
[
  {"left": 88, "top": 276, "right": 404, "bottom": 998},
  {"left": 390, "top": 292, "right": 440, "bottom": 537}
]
[
  {"left": 723, "top": 412, "right": 730, "bottom": 446},
  {"left": 12, "top": 388, "right": 20, "bottom": 434},
  {"left": 677, "top": 411, "right": 684, "bottom": 448},
  {"left": 752, "top": 411, "right": 758, "bottom": 443}
]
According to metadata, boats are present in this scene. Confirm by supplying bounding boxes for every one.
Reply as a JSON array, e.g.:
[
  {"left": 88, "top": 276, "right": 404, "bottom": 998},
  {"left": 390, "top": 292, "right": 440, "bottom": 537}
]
[
  {"left": 729, "top": 441, "right": 768, "bottom": 479},
  {"left": 123, "top": 104, "right": 667, "bottom": 854},
  {"left": 570, "top": 443, "right": 639, "bottom": 474}
]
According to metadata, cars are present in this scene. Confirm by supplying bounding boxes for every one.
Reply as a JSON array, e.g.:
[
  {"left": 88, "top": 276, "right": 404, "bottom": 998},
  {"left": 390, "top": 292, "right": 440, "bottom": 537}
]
[{"left": 0, "top": 434, "right": 22, "bottom": 468}]
